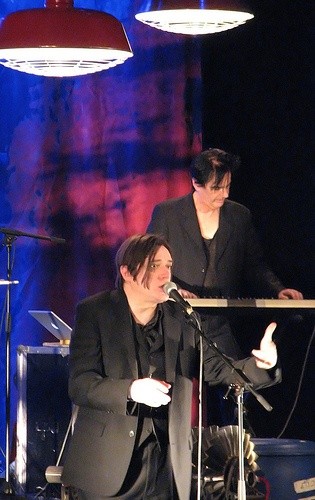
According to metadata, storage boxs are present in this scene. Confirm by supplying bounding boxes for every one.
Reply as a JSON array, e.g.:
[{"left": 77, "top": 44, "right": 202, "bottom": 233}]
[{"left": 249, "top": 437, "right": 315, "bottom": 500}]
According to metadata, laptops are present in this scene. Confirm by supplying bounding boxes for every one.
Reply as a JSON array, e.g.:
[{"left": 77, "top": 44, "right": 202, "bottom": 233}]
[{"left": 27, "top": 310, "right": 72, "bottom": 342}]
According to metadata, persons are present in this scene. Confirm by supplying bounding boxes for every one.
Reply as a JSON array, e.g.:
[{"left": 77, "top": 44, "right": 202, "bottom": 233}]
[
  {"left": 144, "top": 148, "right": 304, "bottom": 428},
  {"left": 59, "top": 233, "right": 283, "bottom": 500}
]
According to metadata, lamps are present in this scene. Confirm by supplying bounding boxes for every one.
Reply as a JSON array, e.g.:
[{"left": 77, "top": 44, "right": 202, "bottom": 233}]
[
  {"left": 136, "top": 0, "right": 254, "bottom": 35},
  {"left": 1, "top": 1, "right": 133, "bottom": 77}
]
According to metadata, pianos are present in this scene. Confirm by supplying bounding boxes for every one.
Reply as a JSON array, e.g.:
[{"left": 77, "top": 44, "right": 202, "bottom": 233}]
[{"left": 170, "top": 293, "right": 315, "bottom": 311}]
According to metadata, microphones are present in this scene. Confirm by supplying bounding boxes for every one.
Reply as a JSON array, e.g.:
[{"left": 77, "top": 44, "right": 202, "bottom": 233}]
[{"left": 162, "top": 282, "right": 198, "bottom": 320}]
